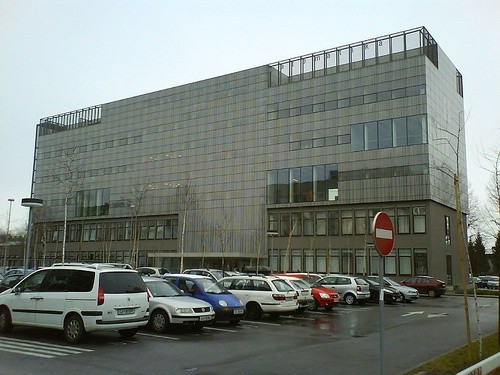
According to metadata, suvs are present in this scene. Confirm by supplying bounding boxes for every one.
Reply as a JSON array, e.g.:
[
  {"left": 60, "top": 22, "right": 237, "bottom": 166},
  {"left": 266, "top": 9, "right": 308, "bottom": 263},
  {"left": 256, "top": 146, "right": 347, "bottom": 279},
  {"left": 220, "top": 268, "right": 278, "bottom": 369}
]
[{"left": 0, "top": 262, "right": 152, "bottom": 346}]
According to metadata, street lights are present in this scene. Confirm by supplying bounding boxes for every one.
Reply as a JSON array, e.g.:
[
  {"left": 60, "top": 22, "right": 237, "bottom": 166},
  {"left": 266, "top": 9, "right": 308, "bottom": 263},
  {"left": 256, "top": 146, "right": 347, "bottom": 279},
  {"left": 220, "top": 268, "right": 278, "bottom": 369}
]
[{"left": 3, "top": 199, "right": 15, "bottom": 273}]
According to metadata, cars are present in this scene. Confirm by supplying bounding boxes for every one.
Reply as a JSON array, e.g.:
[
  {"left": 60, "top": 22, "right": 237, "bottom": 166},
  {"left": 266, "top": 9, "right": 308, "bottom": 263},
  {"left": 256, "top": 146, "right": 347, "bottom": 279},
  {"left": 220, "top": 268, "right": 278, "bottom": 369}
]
[
  {"left": 162, "top": 272, "right": 246, "bottom": 327},
  {"left": 368, "top": 275, "right": 420, "bottom": 303},
  {"left": 345, "top": 274, "right": 400, "bottom": 304},
  {"left": 470, "top": 275, "right": 500, "bottom": 289},
  {"left": 400, "top": 275, "right": 447, "bottom": 298},
  {"left": 140, "top": 275, "right": 216, "bottom": 335},
  {"left": 181, "top": 268, "right": 341, "bottom": 317},
  {"left": 315, "top": 273, "right": 372, "bottom": 305},
  {"left": 0, "top": 265, "right": 44, "bottom": 293},
  {"left": 206, "top": 273, "right": 300, "bottom": 321},
  {"left": 108, "top": 262, "right": 170, "bottom": 278}
]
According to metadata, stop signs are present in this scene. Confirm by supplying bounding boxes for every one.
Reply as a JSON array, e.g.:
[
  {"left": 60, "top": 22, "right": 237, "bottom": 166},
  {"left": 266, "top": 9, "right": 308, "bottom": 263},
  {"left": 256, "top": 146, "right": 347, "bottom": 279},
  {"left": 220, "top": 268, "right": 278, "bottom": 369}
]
[{"left": 373, "top": 211, "right": 396, "bottom": 256}]
